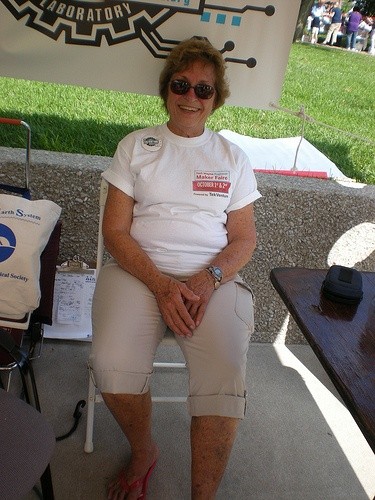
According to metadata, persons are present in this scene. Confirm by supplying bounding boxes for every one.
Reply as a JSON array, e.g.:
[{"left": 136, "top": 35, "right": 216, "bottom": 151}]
[
  {"left": 90, "top": 39, "right": 263, "bottom": 500},
  {"left": 310, "top": 1, "right": 375, "bottom": 53}
]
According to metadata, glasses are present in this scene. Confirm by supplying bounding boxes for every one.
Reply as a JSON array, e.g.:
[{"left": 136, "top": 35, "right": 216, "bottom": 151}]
[{"left": 169, "top": 79, "right": 215, "bottom": 99}]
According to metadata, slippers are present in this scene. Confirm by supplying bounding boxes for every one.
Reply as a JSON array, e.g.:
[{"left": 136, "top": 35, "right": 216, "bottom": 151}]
[{"left": 118, "top": 458, "right": 156, "bottom": 500}]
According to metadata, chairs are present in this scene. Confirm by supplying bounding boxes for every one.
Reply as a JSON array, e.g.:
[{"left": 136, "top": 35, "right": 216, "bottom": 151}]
[
  {"left": 0, "top": 117, "right": 63, "bottom": 500},
  {"left": 84, "top": 177, "right": 187, "bottom": 453}
]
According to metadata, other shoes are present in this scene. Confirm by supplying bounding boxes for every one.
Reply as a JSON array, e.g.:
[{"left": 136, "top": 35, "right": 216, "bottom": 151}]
[{"left": 347, "top": 47, "right": 356, "bottom": 51}]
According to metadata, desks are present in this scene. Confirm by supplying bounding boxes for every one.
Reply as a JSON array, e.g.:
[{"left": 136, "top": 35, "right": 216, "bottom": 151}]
[{"left": 270, "top": 266, "right": 375, "bottom": 457}]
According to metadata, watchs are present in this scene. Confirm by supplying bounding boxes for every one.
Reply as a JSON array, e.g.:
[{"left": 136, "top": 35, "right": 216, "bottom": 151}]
[{"left": 205, "top": 265, "right": 224, "bottom": 290}]
